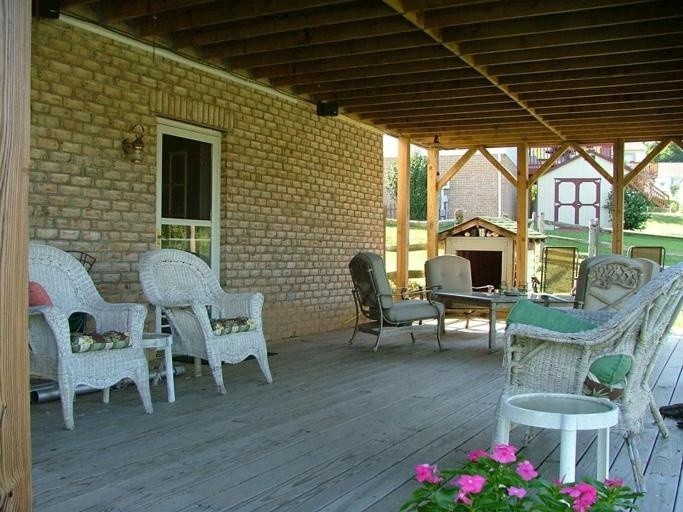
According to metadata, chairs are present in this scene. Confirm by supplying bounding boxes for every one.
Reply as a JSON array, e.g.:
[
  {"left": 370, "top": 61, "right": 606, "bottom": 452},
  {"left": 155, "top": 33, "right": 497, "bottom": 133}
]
[
  {"left": 424, "top": 254, "right": 494, "bottom": 335},
  {"left": 494, "top": 262, "right": 683, "bottom": 495},
  {"left": 139, "top": 248, "right": 273, "bottom": 395},
  {"left": 28, "top": 243, "right": 153, "bottom": 431},
  {"left": 627, "top": 245, "right": 666, "bottom": 270},
  {"left": 531, "top": 245, "right": 579, "bottom": 296},
  {"left": 573, "top": 255, "right": 660, "bottom": 310},
  {"left": 347, "top": 252, "right": 444, "bottom": 352}
]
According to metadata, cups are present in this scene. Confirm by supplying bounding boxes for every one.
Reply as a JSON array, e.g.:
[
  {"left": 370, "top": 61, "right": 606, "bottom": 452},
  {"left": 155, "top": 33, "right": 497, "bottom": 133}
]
[{"left": 478, "top": 228, "right": 486, "bottom": 238}]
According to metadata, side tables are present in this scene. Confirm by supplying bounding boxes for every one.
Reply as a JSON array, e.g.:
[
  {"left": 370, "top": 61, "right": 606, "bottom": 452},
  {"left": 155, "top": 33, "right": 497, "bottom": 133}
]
[
  {"left": 141, "top": 332, "right": 175, "bottom": 402},
  {"left": 495, "top": 392, "right": 619, "bottom": 484}
]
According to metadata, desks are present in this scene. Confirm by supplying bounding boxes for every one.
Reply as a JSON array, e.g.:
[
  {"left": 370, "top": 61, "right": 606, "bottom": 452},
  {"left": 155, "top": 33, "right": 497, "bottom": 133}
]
[{"left": 436, "top": 291, "right": 575, "bottom": 353}]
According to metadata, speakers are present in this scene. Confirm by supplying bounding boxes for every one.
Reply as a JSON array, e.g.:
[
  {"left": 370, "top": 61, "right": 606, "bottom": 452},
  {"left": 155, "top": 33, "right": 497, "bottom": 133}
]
[{"left": 317, "top": 103, "right": 339, "bottom": 116}]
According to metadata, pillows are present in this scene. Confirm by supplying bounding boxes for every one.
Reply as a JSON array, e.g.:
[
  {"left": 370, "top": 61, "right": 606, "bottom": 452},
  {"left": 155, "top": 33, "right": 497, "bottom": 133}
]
[
  {"left": 505, "top": 297, "right": 631, "bottom": 384},
  {"left": 29, "top": 282, "right": 53, "bottom": 304}
]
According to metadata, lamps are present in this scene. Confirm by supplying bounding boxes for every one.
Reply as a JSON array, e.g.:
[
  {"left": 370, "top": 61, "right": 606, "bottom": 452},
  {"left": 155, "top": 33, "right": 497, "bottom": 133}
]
[{"left": 123, "top": 123, "right": 144, "bottom": 163}]
